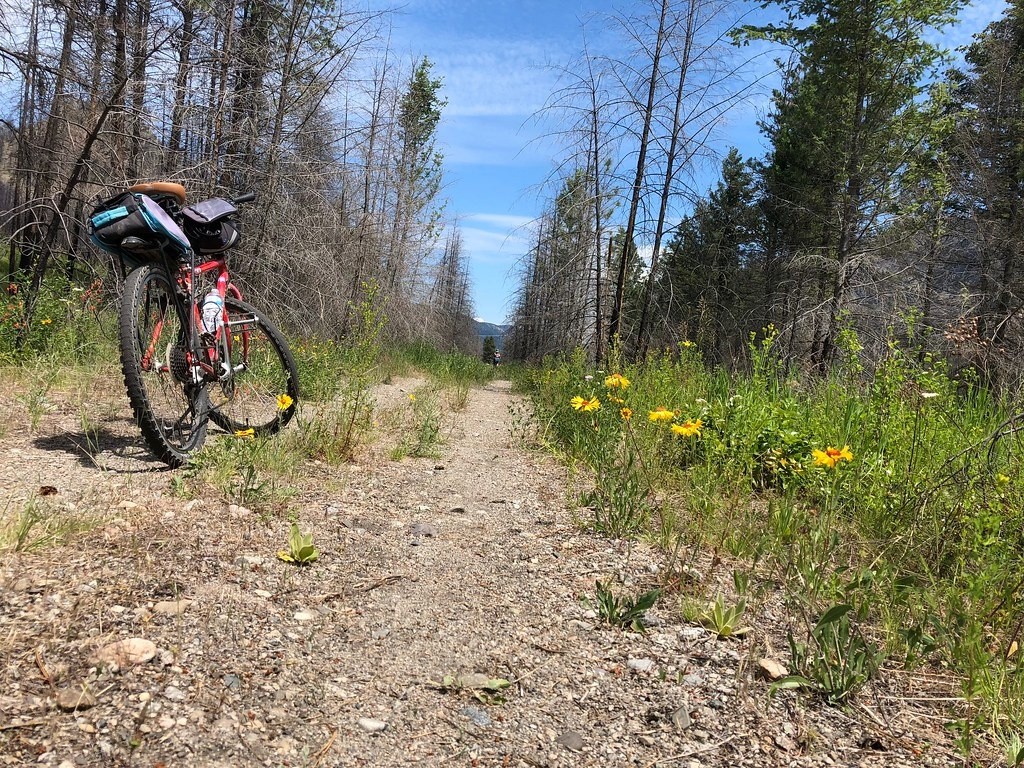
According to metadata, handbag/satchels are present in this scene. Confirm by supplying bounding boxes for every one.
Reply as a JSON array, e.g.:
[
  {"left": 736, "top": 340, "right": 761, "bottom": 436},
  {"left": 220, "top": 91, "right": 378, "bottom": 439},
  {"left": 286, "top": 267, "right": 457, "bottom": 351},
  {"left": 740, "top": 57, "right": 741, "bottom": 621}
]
[{"left": 182, "top": 196, "right": 242, "bottom": 255}]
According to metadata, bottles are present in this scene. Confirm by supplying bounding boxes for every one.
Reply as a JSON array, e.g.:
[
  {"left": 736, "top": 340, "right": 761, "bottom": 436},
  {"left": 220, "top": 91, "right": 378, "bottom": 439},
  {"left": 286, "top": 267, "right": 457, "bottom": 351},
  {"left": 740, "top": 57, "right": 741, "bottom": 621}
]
[{"left": 201, "top": 288, "right": 224, "bottom": 333}]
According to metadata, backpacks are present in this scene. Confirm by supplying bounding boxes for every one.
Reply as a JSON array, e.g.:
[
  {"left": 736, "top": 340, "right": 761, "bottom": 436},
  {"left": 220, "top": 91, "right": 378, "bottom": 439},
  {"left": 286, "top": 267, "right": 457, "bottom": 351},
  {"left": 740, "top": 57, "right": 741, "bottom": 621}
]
[{"left": 85, "top": 191, "right": 190, "bottom": 271}]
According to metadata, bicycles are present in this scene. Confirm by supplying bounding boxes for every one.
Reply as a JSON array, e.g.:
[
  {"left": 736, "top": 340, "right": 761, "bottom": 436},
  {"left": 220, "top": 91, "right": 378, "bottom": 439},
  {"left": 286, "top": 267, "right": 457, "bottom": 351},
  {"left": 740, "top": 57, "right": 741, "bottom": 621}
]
[{"left": 99, "top": 181, "right": 300, "bottom": 469}]
